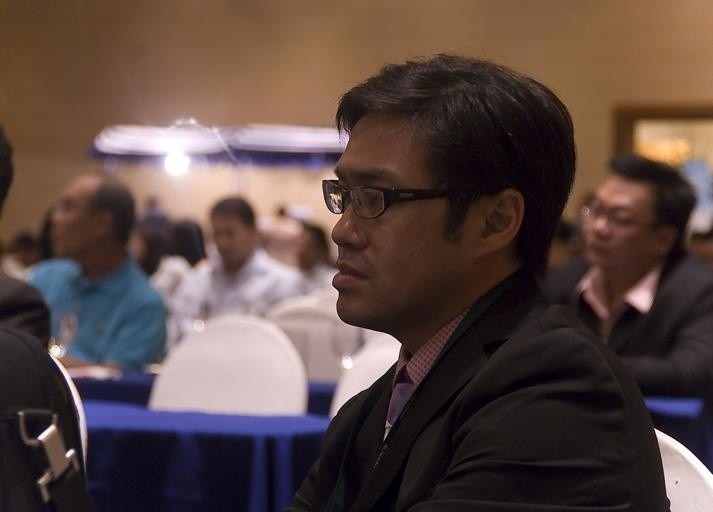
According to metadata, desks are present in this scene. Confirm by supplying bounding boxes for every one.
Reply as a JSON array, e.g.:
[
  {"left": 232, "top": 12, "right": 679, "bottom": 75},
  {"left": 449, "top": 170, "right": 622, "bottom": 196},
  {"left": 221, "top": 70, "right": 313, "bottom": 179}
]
[{"left": 71, "top": 364, "right": 339, "bottom": 504}]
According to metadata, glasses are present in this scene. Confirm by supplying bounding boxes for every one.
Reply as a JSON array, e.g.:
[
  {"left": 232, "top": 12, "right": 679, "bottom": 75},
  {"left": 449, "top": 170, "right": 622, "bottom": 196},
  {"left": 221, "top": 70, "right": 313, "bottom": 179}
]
[{"left": 322, "top": 179, "right": 447, "bottom": 219}]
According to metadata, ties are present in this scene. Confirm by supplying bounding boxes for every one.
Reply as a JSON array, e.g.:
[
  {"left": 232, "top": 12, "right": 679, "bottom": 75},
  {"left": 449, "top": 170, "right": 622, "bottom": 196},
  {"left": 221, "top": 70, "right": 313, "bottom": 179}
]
[{"left": 384, "top": 383, "right": 417, "bottom": 442}]
